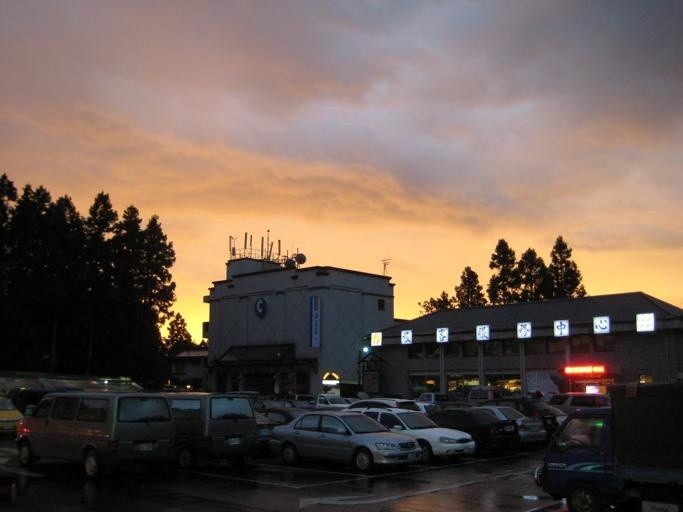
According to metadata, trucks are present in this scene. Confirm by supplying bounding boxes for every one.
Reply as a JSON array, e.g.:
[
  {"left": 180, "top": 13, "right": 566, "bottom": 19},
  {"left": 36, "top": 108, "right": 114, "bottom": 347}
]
[{"left": 608, "top": 377, "right": 683, "bottom": 512}]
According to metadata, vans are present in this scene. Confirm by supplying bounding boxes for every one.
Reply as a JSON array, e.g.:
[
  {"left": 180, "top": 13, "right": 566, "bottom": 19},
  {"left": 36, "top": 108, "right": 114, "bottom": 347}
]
[
  {"left": 137, "top": 393, "right": 260, "bottom": 470},
  {"left": 14, "top": 390, "right": 177, "bottom": 476}
]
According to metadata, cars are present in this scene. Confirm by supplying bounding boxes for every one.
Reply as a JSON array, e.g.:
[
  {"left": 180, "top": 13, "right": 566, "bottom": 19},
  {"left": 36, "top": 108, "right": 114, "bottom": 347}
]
[
  {"left": 425, "top": 409, "right": 523, "bottom": 459},
  {"left": 345, "top": 406, "right": 476, "bottom": 466},
  {"left": 478, "top": 405, "right": 549, "bottom": 448},
  {"left": 515, "top": 401, "right": 569, "bottom": 435},
  {"left": 1, "top": 381, "right": 506, "bottom": 459},
  {"left": 547, "top": 392, "right": 607, "bottom": 419},
  {"left": 268, "top": 410, "right": 424, "bottom": 472}
]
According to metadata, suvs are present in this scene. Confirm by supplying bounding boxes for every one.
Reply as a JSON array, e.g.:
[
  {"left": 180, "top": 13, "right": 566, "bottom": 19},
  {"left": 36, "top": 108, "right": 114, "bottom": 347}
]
[{"left": 534, "top": 407, "right": 611, "bottom": 512}]
